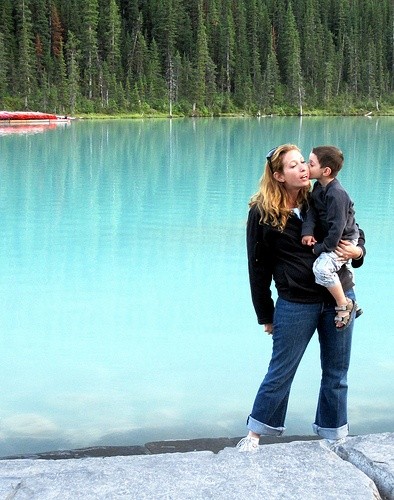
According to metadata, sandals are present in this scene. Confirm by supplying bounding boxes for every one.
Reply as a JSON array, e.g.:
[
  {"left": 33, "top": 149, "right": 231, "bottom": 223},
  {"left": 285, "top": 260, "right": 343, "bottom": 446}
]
[
  {"left": 334, "top": 297, "right": 358, "bottom": 331},
  {"left": 355, "top": 303, "right": 363, "bottom": 319}
]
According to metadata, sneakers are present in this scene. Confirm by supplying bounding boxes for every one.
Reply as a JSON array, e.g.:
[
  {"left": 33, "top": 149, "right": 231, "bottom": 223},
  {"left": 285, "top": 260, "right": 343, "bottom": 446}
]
[{"left": 237, "top": 436, "right": 260, "bottom": 453}]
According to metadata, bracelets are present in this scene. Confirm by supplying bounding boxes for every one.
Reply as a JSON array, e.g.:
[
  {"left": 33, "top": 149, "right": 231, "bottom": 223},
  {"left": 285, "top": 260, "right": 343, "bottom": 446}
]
[{"left": 355, "top": 246, "right": 363, "bottom": 260}]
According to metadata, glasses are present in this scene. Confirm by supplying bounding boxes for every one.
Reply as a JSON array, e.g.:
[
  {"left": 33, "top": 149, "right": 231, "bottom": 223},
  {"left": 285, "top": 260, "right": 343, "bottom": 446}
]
[{"left": 266, "top": 147, "right": 278, "bottom": 174}]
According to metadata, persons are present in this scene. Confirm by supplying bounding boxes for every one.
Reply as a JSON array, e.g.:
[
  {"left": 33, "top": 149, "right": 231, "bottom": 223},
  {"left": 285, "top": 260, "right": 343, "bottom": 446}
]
[
  {"left": 302, "top": 146, "right": 364, "bottom": 332},
  {"left": 237, "top": 143, "right": 366, "bottom": 450}
]
